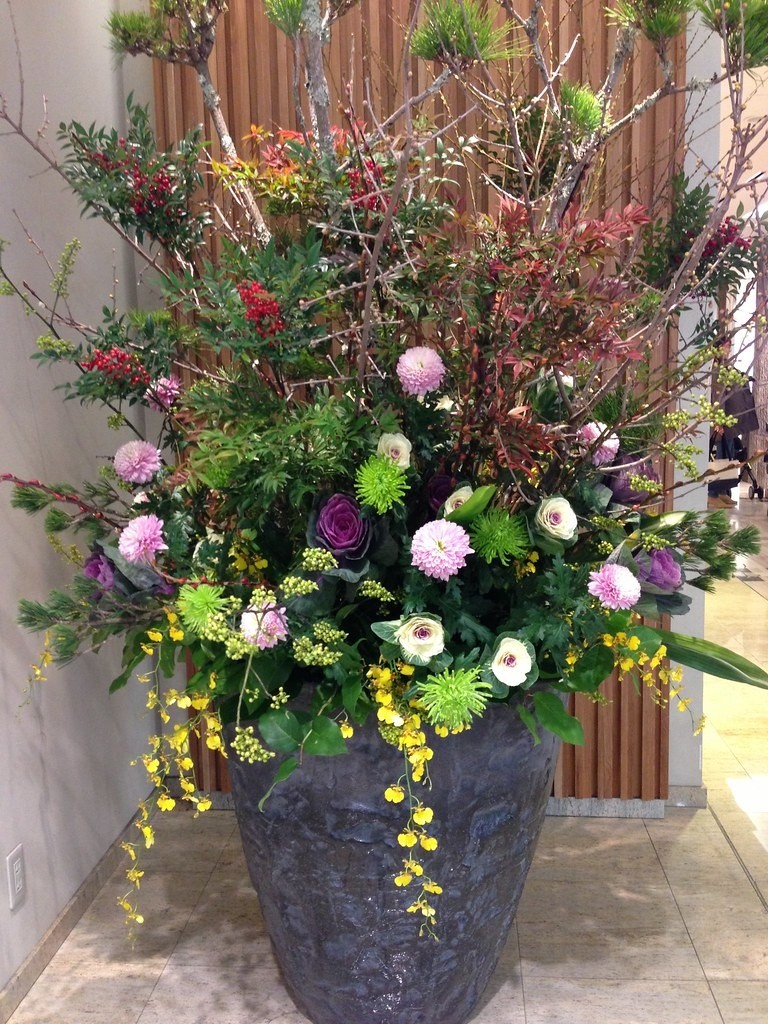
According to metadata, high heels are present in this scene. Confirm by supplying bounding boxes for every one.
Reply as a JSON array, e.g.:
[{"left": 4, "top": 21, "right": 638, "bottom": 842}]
[
  {"left": 708, "top": 496, "right": 732, "bottom": 509},
  {"left": 719, "top": 495, "right": 738, "bottom": 504}
]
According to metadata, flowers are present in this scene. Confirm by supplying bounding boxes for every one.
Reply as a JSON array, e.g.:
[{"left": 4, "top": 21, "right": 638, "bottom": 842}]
[{"left": 0, "top": 0, "right": 768, "bottom": 930}]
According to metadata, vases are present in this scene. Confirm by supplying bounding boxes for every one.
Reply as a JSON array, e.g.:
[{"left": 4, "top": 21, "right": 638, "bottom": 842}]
[{"left": 219, "top": 674, "right": 562, "bottom": 1021}]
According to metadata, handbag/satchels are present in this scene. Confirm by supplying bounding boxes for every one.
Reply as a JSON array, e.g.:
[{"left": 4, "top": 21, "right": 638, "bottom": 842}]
[{"left": 708, "top": 431, "right": 741, "bottom": 490}]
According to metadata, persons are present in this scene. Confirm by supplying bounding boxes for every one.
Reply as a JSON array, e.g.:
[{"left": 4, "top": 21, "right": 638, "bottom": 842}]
[{"left": 708, "top": 336, "right": 759, "bottom": 509}]
